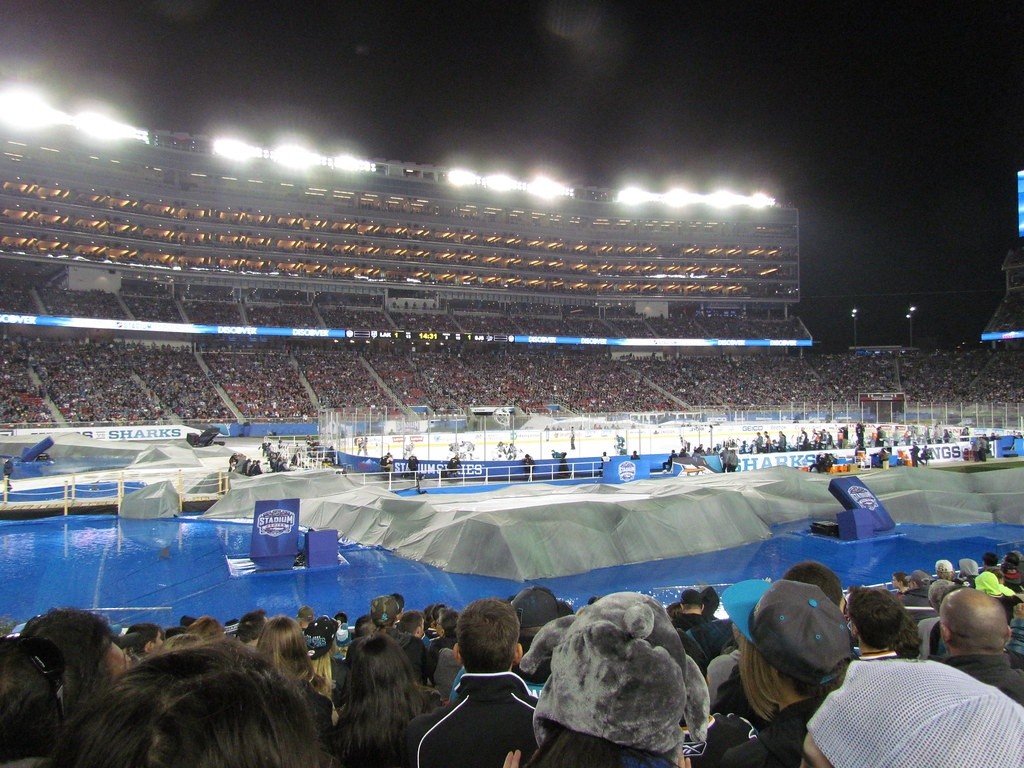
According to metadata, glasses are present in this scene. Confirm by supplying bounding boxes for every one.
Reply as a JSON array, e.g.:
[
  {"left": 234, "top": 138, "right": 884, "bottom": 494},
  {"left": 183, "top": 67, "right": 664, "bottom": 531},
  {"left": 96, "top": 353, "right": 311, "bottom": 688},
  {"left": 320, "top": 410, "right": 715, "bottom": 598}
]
[
  {"left": 0, "top": 632, "right": 65, "bottom": 733},
  {"left": 436, "top": 619, "right": 441, "bottom": 627}
]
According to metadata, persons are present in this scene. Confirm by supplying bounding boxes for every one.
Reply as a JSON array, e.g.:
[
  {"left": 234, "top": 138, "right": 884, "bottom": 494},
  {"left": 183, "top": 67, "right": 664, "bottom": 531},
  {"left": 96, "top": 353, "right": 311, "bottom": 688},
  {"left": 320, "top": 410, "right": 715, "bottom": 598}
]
[
  {"left": 0, "top": 148, "right": 1023, "bottom": 474},
  {"left": 0, "top": 553, "right": 1024, "bottom": 768}
]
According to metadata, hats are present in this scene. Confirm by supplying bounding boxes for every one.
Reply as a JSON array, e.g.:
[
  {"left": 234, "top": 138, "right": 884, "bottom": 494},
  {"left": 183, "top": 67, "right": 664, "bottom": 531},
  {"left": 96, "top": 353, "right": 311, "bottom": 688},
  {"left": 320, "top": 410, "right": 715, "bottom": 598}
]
[
  {"left": 905, "top": 570, "right": 929, "bottom": 585},
  {"left": 225, "top": 618, "right": 239, "bottom": 636},
  {"left": 295, "top": 606, "right": 314, "bottom": 620},
  {"left": 371, "top": 595, "right": 399, "bottom": 626},
  {"left": 806, "top": 659, "right": 1024, "bottom": 768},
  {"left": 681, "top": 589, "right": 702, "bottom": 603},
  {"left": 519, "top": 592, "right": 710, "bottom": 768},
  {"left": 721, "top": 579, "right": 851, "bottom": 684},
  {"left": 303, "top": 615, "right": 338, "bottom": 660},
  {"left": 935, "top": 559, "right": 953, "bottom": 572},
  {"left": 510, "top": 587, "right": 556, "bottom": 637},
  {"left": 1001, "top": 563, "right": 1021, "bottom": 579}
]
